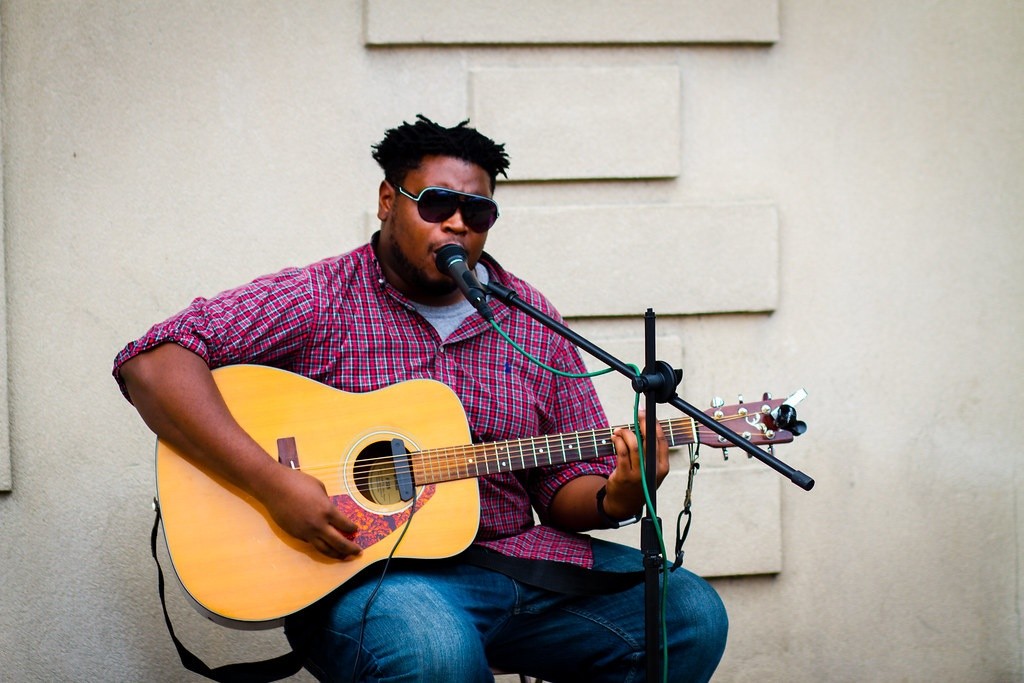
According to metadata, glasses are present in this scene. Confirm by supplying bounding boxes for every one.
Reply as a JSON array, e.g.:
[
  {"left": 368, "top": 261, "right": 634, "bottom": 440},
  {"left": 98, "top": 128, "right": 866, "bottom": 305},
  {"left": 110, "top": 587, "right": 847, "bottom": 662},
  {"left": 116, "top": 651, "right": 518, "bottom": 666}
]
[{"left": 398, "top": 185, "right": 499, "bottom": 233}]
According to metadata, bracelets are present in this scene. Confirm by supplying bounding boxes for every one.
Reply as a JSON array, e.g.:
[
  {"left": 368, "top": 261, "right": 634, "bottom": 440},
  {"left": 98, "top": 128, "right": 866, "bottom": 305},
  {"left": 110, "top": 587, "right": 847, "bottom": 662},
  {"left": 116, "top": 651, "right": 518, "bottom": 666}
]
[{"left": 596, "top": 484, "right": 644, "bottom": 529}]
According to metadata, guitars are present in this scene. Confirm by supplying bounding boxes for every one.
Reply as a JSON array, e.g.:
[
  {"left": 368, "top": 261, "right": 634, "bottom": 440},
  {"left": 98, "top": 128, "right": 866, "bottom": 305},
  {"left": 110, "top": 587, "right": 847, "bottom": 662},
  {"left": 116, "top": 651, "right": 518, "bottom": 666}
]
[{"left": 152, "top": 362, "right": 807, "bottom": 631}]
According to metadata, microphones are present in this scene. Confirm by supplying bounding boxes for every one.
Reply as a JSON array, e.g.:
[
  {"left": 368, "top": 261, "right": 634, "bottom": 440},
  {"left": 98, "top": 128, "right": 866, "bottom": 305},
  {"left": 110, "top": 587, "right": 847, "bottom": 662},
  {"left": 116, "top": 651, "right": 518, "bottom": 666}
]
[{"left": 436, "top": 244, "right": 495, "bottom": 322}]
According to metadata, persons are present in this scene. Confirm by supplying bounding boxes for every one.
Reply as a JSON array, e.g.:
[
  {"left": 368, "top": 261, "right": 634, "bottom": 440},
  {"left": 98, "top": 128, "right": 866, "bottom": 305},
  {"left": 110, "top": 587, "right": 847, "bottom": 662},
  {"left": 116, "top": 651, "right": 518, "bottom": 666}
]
[{"left": 111, "top": 116, "right": 730, "bottom": 683}]
